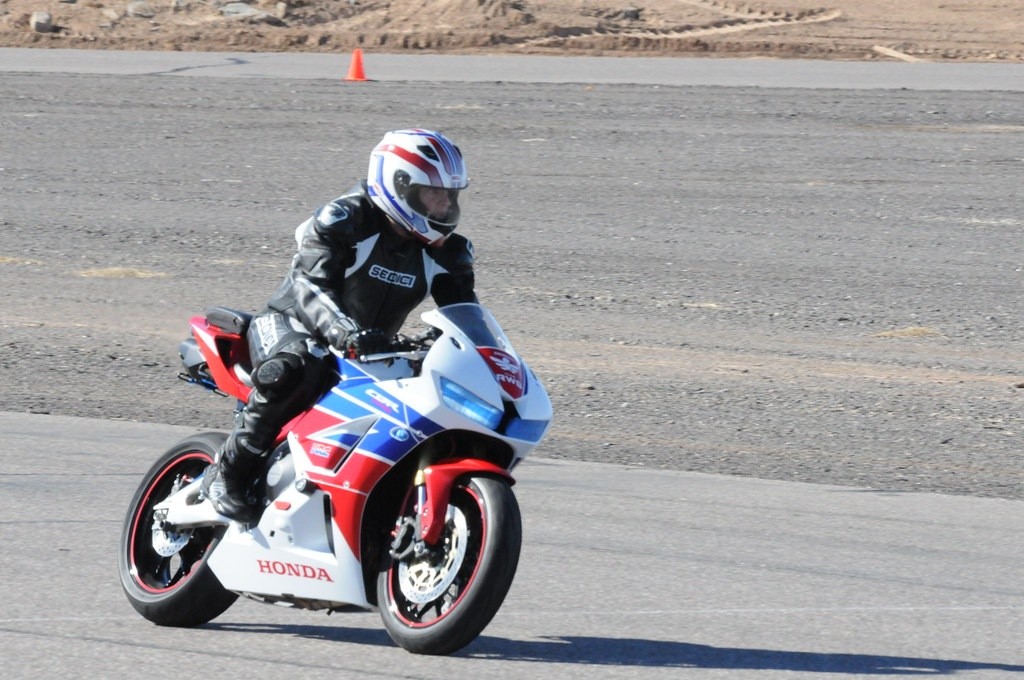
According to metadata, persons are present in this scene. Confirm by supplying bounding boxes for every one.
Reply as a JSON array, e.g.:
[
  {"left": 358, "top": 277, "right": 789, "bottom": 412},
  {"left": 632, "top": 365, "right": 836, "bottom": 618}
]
[{"left": 199, "top": 128, "right": 498, "bottom": 521}]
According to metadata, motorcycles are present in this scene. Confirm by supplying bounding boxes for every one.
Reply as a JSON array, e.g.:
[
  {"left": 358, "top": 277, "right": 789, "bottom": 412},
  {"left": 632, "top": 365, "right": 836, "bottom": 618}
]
[{"left": 117, "top": 299, "right": 553, "bottom": 655}]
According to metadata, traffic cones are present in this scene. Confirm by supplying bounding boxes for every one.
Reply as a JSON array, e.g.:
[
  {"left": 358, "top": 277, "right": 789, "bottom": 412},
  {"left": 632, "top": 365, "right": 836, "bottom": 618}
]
[{"left": 343, "top": 48, "right": 369, "bottom": 81}]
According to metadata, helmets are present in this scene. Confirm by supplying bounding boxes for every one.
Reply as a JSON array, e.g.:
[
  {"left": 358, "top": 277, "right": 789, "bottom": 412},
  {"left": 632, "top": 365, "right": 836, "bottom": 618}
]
[{"left": 366, "top": 127, "right": 468, "bottom": 248}]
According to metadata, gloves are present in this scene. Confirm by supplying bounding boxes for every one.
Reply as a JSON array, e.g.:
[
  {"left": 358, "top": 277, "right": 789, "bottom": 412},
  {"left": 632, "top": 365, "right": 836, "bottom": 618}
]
[{"left": 348, "top": 325, "right": 393, "bottom": 364}]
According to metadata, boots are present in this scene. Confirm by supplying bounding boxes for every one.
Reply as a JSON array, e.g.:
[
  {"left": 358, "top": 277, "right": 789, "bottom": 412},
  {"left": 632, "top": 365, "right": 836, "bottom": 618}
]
[{"left": 202, "top": 406, "right": 281, "bottom": 520}]
